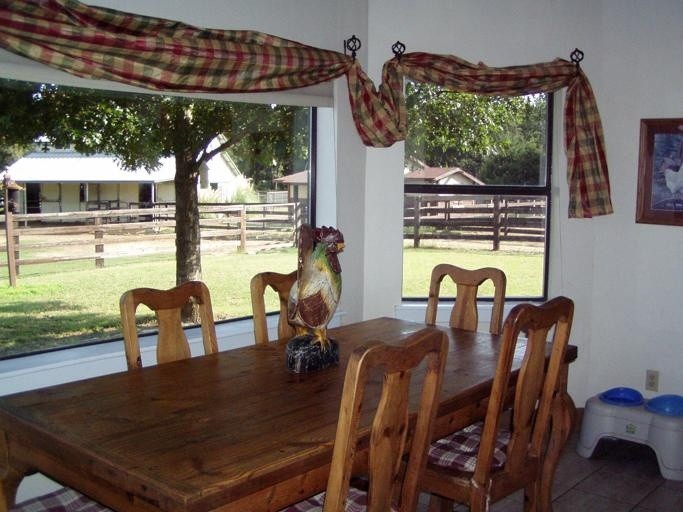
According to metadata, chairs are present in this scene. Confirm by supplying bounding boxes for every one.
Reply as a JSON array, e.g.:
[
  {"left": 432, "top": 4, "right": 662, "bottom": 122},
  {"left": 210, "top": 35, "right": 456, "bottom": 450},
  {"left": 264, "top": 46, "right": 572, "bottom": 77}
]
[
  {"left": 424, "top": 264, "right": 506, "bottom": 336},
  {"left": 119, "top": 281, "right": 218, "bottom": 372},
  {"left": 397, "top": 295, "right": 575, "bottom": 510},
  {"left": 251, "top": 269, "right": 300, "bottom": 343},
  {"left": 278, "top": 327, "right": 449, "bottom": 512}
]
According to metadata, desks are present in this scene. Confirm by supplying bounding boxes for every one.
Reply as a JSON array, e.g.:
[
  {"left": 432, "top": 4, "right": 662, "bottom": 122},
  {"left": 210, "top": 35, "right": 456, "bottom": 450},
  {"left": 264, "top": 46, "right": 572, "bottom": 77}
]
[{"left": 0, "top": 317, "right": 579, "bottom": 510}]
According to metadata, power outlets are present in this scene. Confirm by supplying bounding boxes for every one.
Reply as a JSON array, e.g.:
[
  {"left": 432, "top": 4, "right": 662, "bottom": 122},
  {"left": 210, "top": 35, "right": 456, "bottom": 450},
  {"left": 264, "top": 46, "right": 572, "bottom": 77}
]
[{"left": 647, "top": 369, "right": 658, "bottom": 390}]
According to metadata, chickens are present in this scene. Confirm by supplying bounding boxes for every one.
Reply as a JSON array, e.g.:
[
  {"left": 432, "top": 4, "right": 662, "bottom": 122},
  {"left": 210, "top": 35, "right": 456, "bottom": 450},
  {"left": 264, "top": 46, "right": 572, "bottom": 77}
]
[{"left": 285, "top": 222, "right": 346, "bottom": 352}]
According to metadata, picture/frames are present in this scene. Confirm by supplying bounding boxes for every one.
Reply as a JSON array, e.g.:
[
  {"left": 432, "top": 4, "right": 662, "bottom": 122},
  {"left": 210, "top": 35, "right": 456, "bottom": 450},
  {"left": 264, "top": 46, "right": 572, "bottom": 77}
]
[{"left": 636, "top": 118, "right": 681, "bottom": 229}]
